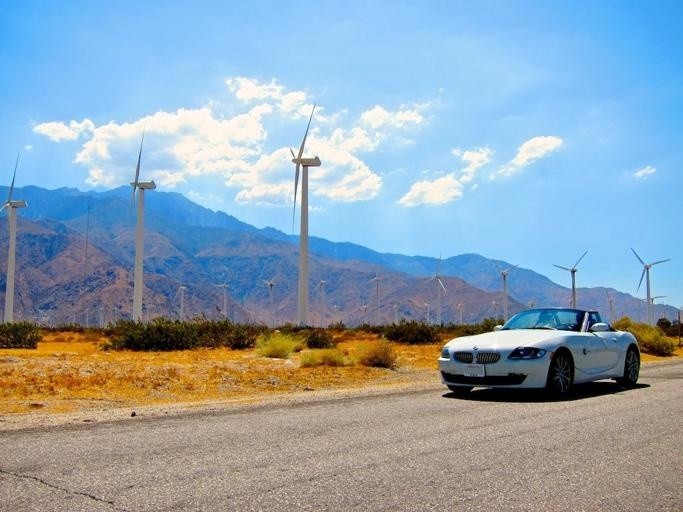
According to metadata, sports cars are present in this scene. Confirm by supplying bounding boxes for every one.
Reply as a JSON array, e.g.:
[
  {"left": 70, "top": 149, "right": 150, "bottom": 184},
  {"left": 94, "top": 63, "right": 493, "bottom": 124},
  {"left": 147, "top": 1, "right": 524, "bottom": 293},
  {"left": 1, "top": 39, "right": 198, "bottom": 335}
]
[{"left": 437, "top": 306, "right": 640, "bottom": 400}]
